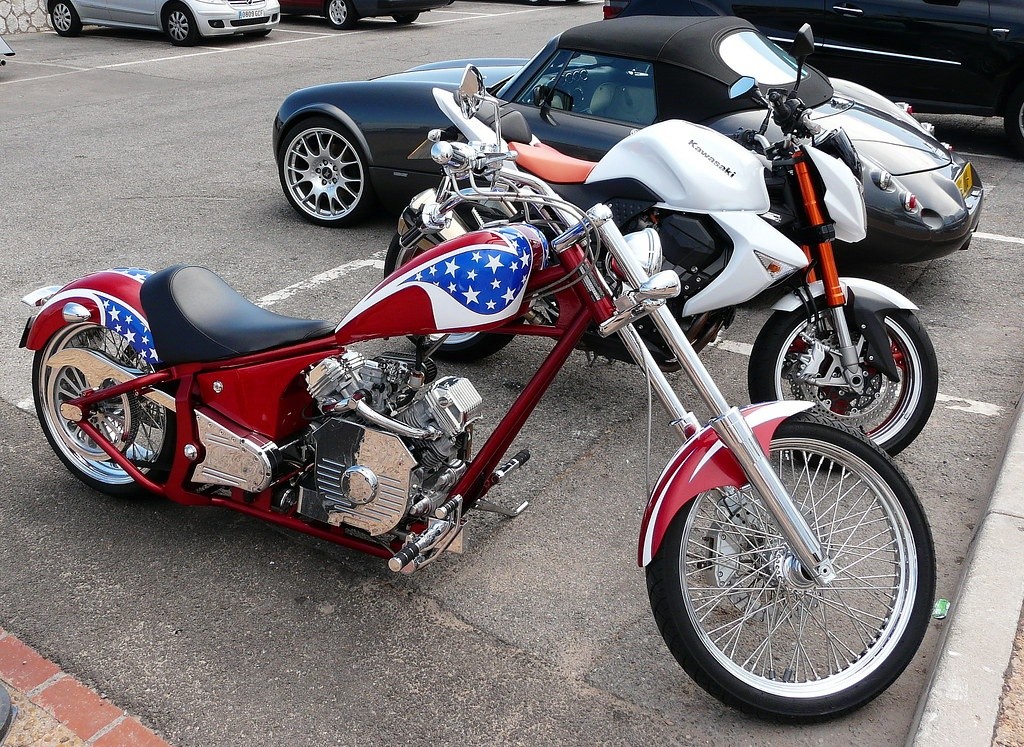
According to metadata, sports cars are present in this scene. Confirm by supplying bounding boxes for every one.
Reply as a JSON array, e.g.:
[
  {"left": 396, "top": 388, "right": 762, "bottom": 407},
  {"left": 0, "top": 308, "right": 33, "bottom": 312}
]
[{"left": 271, "top": 12, "right": 984, "bottom": 284}]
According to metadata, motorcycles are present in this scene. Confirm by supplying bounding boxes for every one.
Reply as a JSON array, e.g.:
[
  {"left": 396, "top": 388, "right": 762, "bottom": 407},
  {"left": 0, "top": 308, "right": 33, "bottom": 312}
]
[
  {"left": 16, "top": 66, "right": 938, "bottom": 726},
  {"left": 383, "top": 22, "right": 940, "bottom": 470}
]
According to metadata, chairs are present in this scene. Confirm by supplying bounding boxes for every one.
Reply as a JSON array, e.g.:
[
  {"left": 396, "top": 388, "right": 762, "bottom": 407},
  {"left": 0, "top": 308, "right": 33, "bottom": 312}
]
[{"left": 589, "top": 81, "right": 636, "bottom": 121}]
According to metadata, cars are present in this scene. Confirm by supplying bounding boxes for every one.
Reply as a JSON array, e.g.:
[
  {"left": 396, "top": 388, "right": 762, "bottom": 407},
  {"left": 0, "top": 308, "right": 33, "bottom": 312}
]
[
  {"left": 47, "top": 0, "right": 280, "bottom": 47},
  {"left": 603, "top": 0, "right": 1024, "bottom": 161},
  {"left": 274, "top": 0, "right": 455, "bottom": 29}
]
